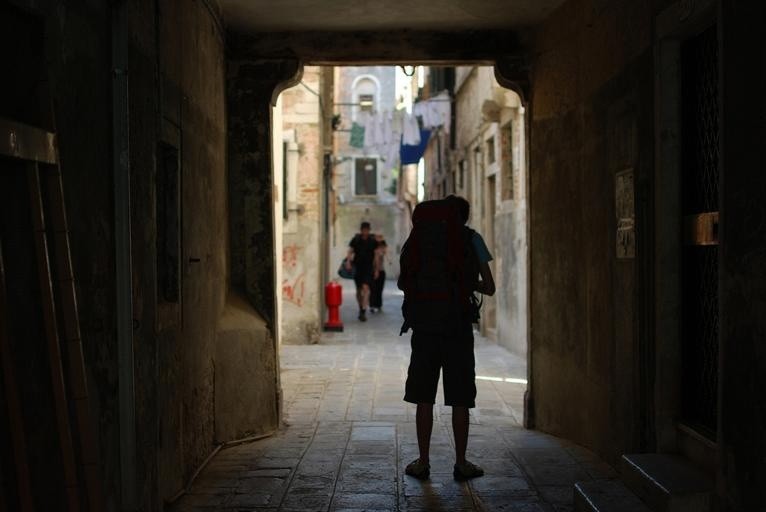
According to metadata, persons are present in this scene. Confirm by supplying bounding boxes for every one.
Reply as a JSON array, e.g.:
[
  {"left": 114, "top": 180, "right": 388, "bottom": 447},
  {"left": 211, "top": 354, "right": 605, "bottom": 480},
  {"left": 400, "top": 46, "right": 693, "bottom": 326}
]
[
  {"left": 397, "top": 195, "right": 496, "bottom": 482},
  {"left": 344, "top": 221, "right": 392, "bottom": 322}
]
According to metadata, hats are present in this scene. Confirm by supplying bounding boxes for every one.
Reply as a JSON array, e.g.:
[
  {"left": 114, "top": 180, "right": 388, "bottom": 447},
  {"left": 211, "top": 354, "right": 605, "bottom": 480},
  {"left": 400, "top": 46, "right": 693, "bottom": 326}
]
[{"left": 374, "top": 230, "right": 386, "bottom": 241}]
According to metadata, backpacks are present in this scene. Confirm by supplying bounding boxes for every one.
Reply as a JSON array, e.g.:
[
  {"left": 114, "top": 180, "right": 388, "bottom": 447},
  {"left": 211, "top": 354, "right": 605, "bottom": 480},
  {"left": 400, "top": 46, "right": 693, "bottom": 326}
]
[{"left": 400, "top": 199, "right": 480, "bottom": 332}]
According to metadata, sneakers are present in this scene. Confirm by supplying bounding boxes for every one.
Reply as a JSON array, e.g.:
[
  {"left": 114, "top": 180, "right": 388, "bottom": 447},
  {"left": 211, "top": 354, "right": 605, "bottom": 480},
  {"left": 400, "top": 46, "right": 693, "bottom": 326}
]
[
  {"left": 405, "top": 457, "right": 430, "bottom": 480},
  {"left": 453, "top": 460, "right": 484, "bottom": 482},
  {"left": 359, "top": 311, "right": 367, "bottom": 321}
]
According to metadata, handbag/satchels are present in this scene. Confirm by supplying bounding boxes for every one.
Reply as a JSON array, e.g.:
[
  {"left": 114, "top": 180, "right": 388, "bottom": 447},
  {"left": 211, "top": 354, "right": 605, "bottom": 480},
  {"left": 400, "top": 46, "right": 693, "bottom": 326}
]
[{"left": 338, "top": 258, "right": 356, "bottom": 278}]
